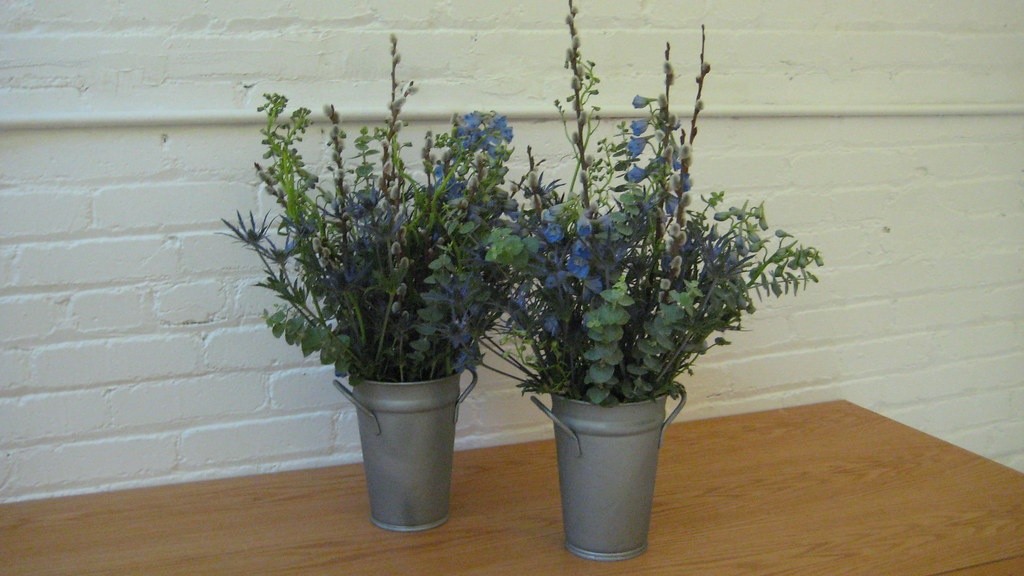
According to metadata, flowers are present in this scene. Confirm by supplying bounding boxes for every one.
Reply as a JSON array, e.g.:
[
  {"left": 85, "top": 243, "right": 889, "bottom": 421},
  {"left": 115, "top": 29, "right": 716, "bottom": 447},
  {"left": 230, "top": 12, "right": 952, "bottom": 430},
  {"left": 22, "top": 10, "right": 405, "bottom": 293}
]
[
  {"left": 220, "top": 0, "right": 533, "bottom": 383},
  {"left": 489, "top": 0, "right": 821, "bottom": 406}
]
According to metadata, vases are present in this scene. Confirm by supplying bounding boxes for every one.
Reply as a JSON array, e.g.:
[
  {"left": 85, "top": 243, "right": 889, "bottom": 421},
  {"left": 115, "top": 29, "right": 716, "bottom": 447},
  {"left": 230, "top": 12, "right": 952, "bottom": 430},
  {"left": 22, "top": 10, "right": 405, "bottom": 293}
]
[
  {"left": 331, "top": 366, "right": 478, "bottom": 532},
  {"left": 531, "top": 387, "right": 687, "bottom": 561}
]
